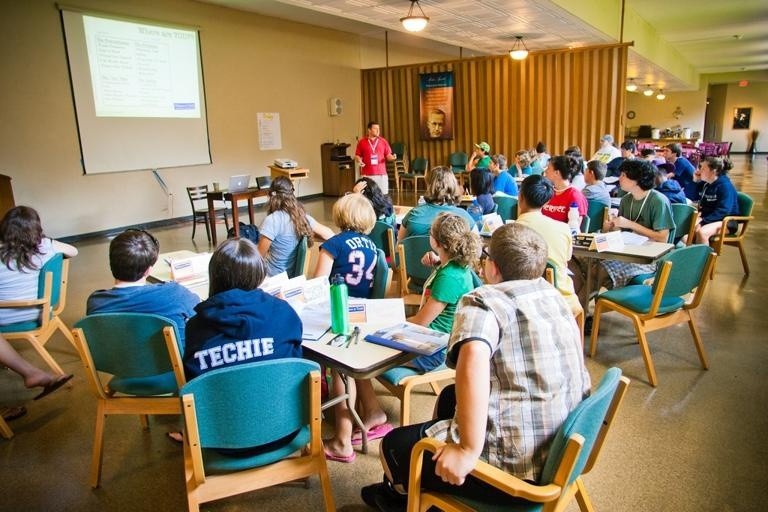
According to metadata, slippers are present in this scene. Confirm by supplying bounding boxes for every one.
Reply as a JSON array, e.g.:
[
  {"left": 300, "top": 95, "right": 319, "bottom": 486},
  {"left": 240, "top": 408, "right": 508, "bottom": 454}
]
[
  {"left": 31, "top": 373, "right": 74, "bottom": 401},
  {"left": 351, "top": 422, "right": 394, "bottom": 445},
  {"left": 0, "top": 406, "right": 28, "bottom": 422},
  {"left": 321, "top": 439, "right": 356, "bottom": 464}
]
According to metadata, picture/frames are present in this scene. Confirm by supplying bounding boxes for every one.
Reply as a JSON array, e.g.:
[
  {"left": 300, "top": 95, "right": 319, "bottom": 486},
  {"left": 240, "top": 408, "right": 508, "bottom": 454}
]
[{"left": 731, "top": 106, "right": 752, "bottom": 130}]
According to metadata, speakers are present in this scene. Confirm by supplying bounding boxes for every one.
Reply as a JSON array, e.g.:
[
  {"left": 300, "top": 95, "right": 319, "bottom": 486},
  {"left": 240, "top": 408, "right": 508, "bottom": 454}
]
[{"left": 331, "top": 97, "right": 343, "bottom": 116}]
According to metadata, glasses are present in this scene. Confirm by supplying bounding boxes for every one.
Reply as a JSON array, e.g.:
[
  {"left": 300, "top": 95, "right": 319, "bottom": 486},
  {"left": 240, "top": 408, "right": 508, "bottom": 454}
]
[{"left": 481, "top": 245, "right": 491, "bottom": 261}]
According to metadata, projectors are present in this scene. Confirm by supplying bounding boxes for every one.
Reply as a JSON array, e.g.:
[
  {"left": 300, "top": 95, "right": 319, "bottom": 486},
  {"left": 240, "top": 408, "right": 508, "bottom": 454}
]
[{"left": 274, "top": 158, "right": 298, "bottom": 168}]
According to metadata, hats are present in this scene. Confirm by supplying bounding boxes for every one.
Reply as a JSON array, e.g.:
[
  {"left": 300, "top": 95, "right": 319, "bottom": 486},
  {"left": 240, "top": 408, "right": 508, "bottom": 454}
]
[
  {"left": 600, "top": 135, "right": 613, "bottom": 144},
  {"left": 473, "top": 141, "right": 490, "bottom": 154}
]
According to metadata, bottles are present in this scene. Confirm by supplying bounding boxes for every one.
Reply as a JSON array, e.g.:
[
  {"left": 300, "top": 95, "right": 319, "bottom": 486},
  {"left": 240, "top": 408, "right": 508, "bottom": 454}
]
[
  {"left": 418, "top": 195, "right": 427, "bottom": 206},
  {"left": 330, "top": 273, "right": 352, "bottom": 333},
  {"left": 568, "top": 202, "right": 581, "bottom": 244},
  {"left": 468, "top": 200, "right": 483, "bottom": 231}
]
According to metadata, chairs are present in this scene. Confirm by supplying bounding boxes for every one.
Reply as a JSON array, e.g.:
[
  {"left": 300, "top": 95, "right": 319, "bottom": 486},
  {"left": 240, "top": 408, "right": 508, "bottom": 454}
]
[
  {"left": 406, "top": 368, "right": 630, "bottom": 512},
  {"left": 178, "top": 357, "right": 335, "bottom": 512},
  {"left": 382, "top": 141, "right": 470, "bottom": 194},
  {"left": 379, "top": 195, "right": 485, "bottom": 425},
  {"left": 187, "top": 184, "right": 229, "bottom": 243},
  {"left": 635, "top": 142, "right": 733, "bottom": 171},
  {"left": 545, "top": 261, "right": 584, "bottom": 348},
  {"left": 588, "top": 243, "right": 718, "bottom": 388},
  {"left": 669, "top": 190, "right": 753, "bottom": 280},
  {"left": 72, "top": 312, "right": 186, "bottom": 488},
  {"left": 492, "top": 196, "right": 674, "bottom": 312},
  {"left": 0, "top": 250, "right": 79, "bottom": 391}
]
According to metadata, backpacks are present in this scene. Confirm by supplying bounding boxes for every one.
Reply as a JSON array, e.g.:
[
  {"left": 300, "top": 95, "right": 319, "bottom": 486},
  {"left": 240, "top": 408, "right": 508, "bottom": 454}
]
[{"left": 226, "top": 220, "right": 261, "bottom": 246}]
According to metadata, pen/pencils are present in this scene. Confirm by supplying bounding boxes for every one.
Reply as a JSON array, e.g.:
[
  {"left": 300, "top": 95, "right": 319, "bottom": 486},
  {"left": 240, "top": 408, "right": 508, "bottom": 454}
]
[{"left": 346, "top": 327, "right": 359, "bottom": 349}]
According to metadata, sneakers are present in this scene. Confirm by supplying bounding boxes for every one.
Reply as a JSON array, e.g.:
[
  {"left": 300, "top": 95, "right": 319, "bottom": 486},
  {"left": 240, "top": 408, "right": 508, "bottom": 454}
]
[
  {"left": 584, "top": 315, "right": 600, "bottom": 336},
  {"left": 360, "top": 475, "right": 409, "bottom": 512}
]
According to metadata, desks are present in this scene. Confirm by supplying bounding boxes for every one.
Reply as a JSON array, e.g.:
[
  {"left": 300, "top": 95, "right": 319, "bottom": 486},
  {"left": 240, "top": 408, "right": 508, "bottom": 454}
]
[
  {"left": 206, "top": 186, "right": 272, "bottom": 248},
  {"left": 147, "top": 250, "right": 441, "bottom": 455}
]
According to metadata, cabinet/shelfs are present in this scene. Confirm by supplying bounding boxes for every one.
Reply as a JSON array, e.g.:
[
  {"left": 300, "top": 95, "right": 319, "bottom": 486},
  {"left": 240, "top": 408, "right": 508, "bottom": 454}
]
[{"left": 321, "top": 143, "right": 356, "bottom": 197}]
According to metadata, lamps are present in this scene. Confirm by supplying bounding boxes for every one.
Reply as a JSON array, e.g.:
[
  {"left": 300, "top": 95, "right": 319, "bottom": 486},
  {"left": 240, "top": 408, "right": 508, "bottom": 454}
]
[
  {"left": 656, "top": 89, "right": 665, "bottom": 101},
  {"left": 626, "top": 77, "right": 637, "bottom": 93},
  {"left": 643, "top": 84, "right": 655, "bottom": 96},
  {"left": 399, "top": 0, "right": 429, "bottom": 33},
  {"left": 507, "top": 34, "right": 528, "bottom": 60}
]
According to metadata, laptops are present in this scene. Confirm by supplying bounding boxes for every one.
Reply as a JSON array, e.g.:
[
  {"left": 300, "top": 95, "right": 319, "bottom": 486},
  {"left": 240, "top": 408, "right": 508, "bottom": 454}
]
[{"left": 227, "top": 175, "right": 251, "bottom": 193}]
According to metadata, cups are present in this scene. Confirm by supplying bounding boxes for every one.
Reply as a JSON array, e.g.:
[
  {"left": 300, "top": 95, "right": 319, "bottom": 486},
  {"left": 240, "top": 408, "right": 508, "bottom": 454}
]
[{"left": 213, "top": 182, "right": 220, "bottom": 193}]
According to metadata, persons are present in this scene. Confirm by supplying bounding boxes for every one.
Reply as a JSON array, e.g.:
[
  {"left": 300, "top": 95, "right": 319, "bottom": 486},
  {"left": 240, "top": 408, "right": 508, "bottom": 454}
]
[
  {"left": 0, "top": 207, "right": 79, "bottom": 326},
  {"left": 0, "top": 331, "right": 75, "bottom": 421},
  {"left": 424, "top": 110, "right": 450, "bottom": 140},
  {"left": 84, "top": 228, "right": 198, "bottom": 397},
  {"left": 165, "top": 238, "right": 302, "bottom": 457}
]
